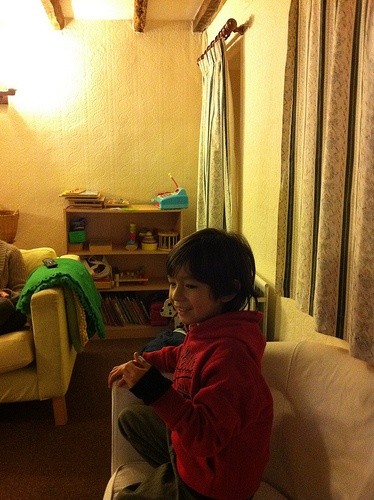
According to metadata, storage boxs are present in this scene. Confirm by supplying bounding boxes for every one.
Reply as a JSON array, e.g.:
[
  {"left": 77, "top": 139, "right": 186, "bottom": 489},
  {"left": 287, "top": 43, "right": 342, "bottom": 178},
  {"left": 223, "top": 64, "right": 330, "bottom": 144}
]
[
  {"left": 89, "top": 238, "right": 112, "bottom": 253},
  {"left": 150, "top": 302, "right": 168, "bottom": 327}
]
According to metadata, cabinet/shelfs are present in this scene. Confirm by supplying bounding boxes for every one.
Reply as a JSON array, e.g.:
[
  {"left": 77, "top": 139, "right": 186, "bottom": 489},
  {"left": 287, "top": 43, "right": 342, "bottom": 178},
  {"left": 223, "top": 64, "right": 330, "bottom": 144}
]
[{"left": 64, "top": 200, "right": 183, "bottom": 341}]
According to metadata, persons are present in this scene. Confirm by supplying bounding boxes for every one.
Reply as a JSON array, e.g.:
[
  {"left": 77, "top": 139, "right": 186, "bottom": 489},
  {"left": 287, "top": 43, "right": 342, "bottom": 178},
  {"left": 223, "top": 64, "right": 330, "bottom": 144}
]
[
  {"left": 0, "top": 240, "right": 27, "bottom": 334},
  {"left": 108, "top": 228, "right": 273, "bottom": 500}
]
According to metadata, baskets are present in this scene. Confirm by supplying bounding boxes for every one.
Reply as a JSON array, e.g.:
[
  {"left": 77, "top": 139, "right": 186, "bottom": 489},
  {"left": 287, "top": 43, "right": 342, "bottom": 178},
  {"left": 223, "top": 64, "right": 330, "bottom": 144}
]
[{"left": 0, "top": 209, "right": 19, "bottom": 243}]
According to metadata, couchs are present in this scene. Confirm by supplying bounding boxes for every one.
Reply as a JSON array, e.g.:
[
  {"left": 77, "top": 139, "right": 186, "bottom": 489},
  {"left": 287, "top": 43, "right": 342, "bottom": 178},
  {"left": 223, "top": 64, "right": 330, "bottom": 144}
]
[{"left": 107, "top": 331, "right": 374, "bottom": 500}]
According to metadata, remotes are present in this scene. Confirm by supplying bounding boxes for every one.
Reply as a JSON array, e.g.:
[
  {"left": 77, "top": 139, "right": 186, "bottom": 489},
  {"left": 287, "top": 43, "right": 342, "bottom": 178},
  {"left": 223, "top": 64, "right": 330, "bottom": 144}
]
[{"left": 42, "top": 257, "right": 58, "bottom": 268}]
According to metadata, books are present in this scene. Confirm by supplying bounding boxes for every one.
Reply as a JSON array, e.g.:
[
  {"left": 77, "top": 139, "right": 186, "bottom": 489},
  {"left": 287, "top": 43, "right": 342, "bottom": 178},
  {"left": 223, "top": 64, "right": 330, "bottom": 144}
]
[{"left": 102, "top": 297, "right": 150, "bottom": 327}]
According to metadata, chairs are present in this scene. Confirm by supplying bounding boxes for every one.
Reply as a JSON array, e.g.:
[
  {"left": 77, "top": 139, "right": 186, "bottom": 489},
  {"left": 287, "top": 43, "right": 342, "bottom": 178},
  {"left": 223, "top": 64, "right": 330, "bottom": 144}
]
[{"left": 0, "top": 246, "right": 81, "bottom": 426}]
